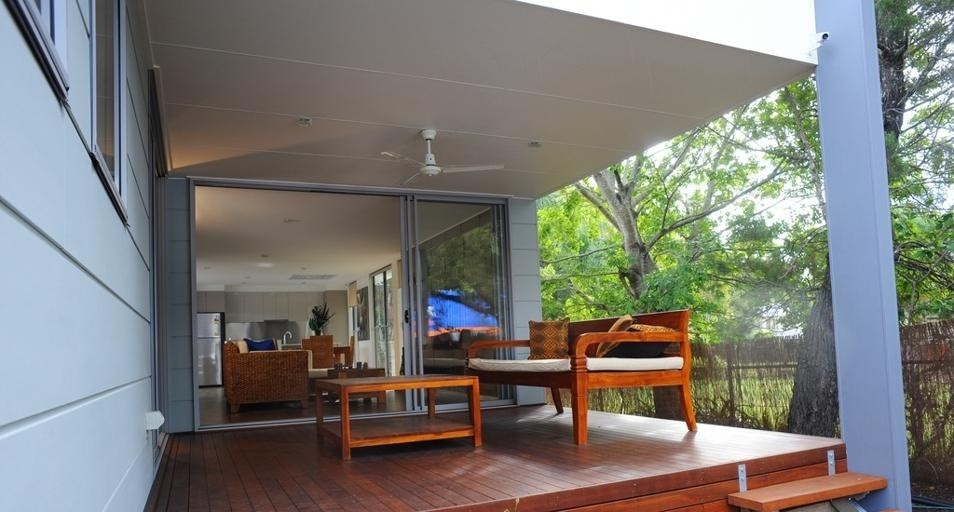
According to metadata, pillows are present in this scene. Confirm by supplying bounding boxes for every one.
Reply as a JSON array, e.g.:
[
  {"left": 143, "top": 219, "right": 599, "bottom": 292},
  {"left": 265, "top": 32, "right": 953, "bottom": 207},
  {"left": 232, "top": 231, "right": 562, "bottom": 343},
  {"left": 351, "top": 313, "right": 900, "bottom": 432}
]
[
  {"left": 243, "top": 337, "right": 276, "bottom": 351},
  {"left": 599, "top": 321, "right": 676, "bottom": 360},
  {"left": 523, "top": 316, "right": 572, "bottom": 359}
]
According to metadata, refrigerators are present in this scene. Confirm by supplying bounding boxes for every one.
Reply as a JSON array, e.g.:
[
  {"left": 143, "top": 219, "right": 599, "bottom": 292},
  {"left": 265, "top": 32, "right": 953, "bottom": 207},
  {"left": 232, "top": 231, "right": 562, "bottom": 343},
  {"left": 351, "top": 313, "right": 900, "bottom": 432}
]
[{"left": 197, "top": 313, "right": 223, "bottom": 386}]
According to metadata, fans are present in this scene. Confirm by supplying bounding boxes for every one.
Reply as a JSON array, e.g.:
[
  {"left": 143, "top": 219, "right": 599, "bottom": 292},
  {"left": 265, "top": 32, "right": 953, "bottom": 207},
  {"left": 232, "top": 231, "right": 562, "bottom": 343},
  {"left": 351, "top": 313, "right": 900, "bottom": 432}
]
[{"left": 379, "top": 129, "right": 504, "bottom": 186}]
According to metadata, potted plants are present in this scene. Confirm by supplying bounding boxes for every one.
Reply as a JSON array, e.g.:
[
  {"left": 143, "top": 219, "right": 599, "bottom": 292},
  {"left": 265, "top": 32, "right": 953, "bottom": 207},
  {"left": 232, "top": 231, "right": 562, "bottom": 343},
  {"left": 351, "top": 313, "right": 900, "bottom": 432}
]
[{"left": 307, "top": 304, "right": 335, "bottom": 335}]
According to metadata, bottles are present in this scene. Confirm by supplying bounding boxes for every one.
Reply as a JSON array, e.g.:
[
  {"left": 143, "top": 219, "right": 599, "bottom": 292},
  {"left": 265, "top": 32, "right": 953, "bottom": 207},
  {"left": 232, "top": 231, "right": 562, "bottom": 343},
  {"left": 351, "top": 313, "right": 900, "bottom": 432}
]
[{"left": 334, "top": 362, "right": 368, "bottom": 370}]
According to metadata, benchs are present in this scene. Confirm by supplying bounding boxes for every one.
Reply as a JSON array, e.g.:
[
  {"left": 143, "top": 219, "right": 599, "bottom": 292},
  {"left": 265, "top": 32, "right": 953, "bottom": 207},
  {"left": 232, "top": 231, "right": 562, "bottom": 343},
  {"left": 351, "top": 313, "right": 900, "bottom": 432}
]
[{"left": 464, "top": 308, "right": 698, "bottom": 449}]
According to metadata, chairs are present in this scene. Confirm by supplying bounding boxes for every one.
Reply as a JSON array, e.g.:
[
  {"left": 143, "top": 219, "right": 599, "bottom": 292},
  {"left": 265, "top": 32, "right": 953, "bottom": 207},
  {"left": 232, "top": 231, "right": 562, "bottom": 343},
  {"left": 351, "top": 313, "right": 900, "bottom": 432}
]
[
  {"left": 301, "top": 335, "right": 355, "bottom": 369},
  {"left": 221, "top": 340, "right": 309, "bottom": 414}
]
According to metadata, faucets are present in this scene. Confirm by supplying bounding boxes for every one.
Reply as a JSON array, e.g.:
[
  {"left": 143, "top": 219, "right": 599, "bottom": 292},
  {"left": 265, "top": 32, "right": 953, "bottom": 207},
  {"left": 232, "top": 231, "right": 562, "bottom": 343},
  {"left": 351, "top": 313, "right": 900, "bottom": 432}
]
[{"left": 283, "top": 332, "right": 292, "bottom": 343}]
